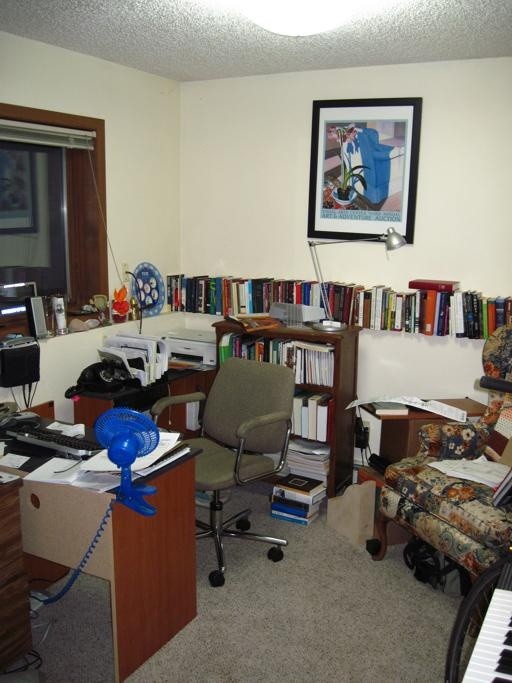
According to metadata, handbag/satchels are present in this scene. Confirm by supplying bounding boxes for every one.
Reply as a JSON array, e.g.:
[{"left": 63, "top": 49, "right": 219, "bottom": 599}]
[{"left": 403, "top": 535, "right": 479, "bottom": 597}]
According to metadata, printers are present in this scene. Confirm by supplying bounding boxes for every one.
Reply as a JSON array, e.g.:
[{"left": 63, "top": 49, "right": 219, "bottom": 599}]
[{"left": 162, "top": 328, "right": 218, "bottom": 367}]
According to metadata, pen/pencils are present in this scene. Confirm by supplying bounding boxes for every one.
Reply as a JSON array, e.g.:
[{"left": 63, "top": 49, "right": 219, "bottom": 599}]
[{"left": 308, "top": 318, "right": 330, "bottom": 324}]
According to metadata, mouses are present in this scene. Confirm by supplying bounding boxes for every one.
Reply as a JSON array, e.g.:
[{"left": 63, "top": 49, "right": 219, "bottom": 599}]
[{"left": 15, "top": 421, "right": 40, "bottom": 428}]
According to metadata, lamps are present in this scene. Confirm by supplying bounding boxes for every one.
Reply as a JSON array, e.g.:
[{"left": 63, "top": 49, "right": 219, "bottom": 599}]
[{"left": 306, "top": 227, "right": 407, "bottom": 330}]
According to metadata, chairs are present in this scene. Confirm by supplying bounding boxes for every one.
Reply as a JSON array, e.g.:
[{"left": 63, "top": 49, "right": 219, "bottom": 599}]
[
  {"left": 151, "top": 355, "right": 297, "bottom": 590},
  {"left": 366, "top": 326, "right": 511, "bottom": 590}
]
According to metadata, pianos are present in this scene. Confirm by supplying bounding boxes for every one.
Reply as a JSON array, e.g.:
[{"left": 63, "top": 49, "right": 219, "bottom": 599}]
[{"left": 460, "top": 588, "right": 512, "bottom": 682}]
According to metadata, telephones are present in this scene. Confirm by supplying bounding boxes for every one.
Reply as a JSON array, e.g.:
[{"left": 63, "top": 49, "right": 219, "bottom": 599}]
[{"left": 77, "top": 362, "right": 122, "bottom": 392}]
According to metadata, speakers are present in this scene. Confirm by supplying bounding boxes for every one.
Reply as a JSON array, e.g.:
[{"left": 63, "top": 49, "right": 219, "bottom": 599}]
[
  {"left": 24, "top": 296, "right": 48, "bottom": 338},
  {"left": 0, "top": 342, "right": 40, "bottom": 387}
]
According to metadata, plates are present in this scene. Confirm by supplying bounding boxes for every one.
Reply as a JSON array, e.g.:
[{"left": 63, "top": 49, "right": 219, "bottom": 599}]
[
  {"left": 131, "top": 263, "right": 165, "bottom": 317},
  {"left": 312, "top": 322, "right": 348, "bottom": 332}
]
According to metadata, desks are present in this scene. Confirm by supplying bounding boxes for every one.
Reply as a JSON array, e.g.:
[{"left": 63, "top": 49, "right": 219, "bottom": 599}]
[
  {"left": 360, "top": 398, "right": 491, "bottom": 462},
  {"left": 1, "top": 417, "right": 203, "bottom": 683}
]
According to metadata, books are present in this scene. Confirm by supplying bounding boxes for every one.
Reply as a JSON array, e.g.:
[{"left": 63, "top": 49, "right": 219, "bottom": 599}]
[
  {"left": 167, "top": 274, "right": 511, "bottom": 341},
  {"left": 371, "top": 402, "right": 408, "bottom": 415},
  {"left": 98, "top": 332, "right": 169, "bottom": 387},
  {"left": 219, "top": 333, "right": 335, "bottom": 525},
  {"left": 491, "top": 470, "right": 511, "bottom": 507},
  {"left": 134, "top": 427, "right": 190, "bottom": 477}
]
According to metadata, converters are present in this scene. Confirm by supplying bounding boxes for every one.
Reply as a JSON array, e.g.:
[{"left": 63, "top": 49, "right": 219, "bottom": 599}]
[
  {"left": 368, "top": 453, "right": 390, "bottom": 475},
  {"left": 354, "top": 430, "right": 369, "bottom": 449}
]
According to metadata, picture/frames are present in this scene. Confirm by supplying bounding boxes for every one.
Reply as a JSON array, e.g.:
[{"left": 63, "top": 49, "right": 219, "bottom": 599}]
[{"left": 306, "top": 98, "right": 421, "bottom": 245}]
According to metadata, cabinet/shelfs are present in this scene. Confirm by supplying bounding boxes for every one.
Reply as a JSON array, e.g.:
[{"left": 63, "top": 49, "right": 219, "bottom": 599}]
[{"left": 73, "top": 321, "right": 360, "bottom": 499}]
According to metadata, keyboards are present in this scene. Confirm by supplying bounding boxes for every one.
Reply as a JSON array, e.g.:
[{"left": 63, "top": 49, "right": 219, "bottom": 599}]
[{"left": 6, "top": 426, "right": 105, "bottom": 457}]
